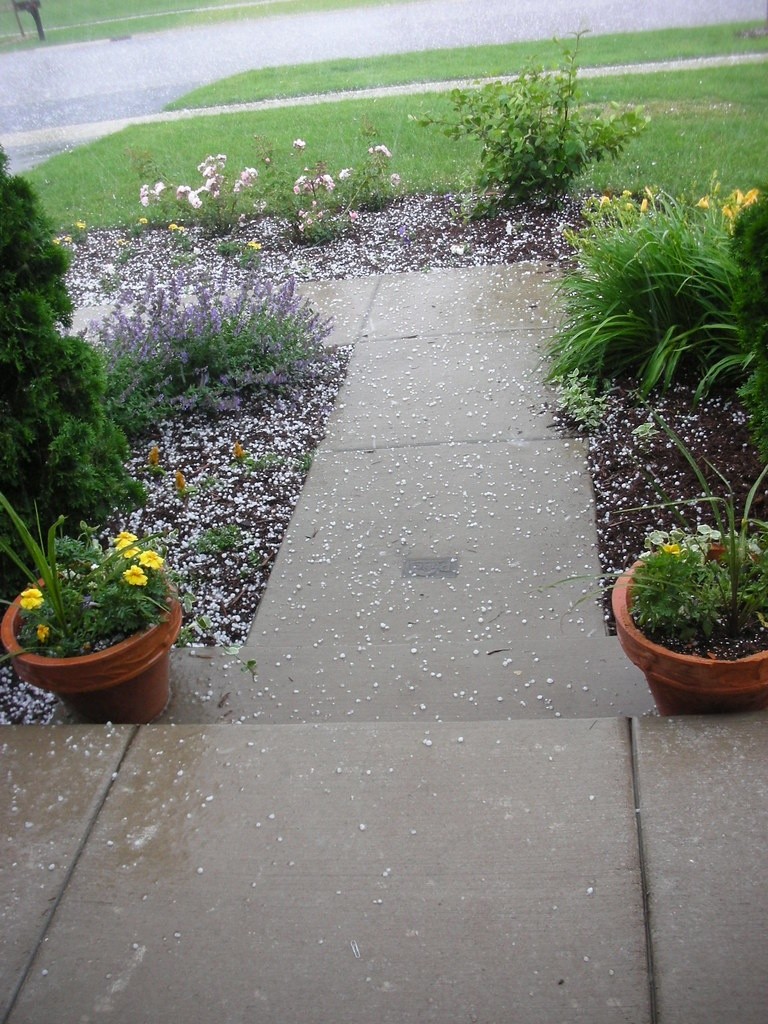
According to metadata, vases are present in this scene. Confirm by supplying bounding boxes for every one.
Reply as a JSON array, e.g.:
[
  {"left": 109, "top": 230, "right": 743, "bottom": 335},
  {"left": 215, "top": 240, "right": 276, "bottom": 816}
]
[
  {"left": 0, "top": 574, "right": 182, "bottom": 725},
  {"left": 612, "top": 542, "right": 768, "bottom": 716}
]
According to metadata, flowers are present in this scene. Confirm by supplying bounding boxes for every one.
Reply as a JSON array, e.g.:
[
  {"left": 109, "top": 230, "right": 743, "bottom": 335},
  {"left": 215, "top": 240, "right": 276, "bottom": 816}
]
[
  {"left": 548, "top": 401, "right": 768, "bottom": 646},
  {"left": 0, "top": 490, "right": 185, "bottom": 666}
]
